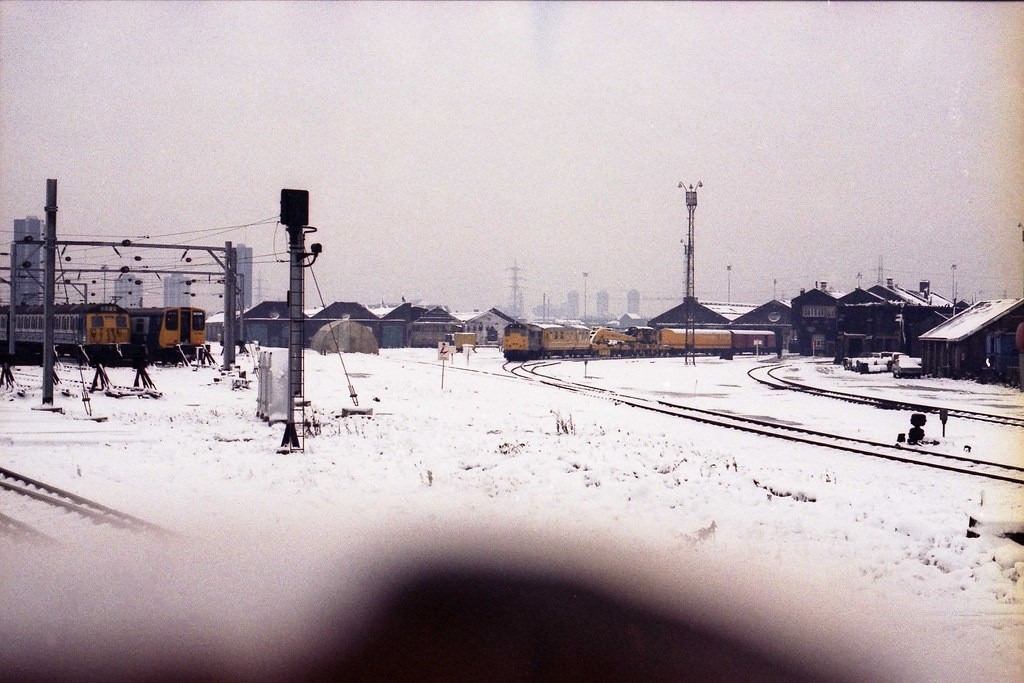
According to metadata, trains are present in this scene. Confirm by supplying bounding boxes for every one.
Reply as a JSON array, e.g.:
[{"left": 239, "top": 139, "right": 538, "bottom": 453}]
[
  {"left": 0, "top": 301, "right": 132, "bottom": 357},
  {"left": 500, "top": 319, "right": 777, "bottom": 362},
  {"left": 126, "top": 307, "right": 206, "bottom": 364}
]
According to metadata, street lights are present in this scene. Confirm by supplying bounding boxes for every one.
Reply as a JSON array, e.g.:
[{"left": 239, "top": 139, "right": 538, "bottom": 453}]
[
  {"left": 726, "top": 264, "right": 734, "bottom": 304},
  {"left": 583, "top": 272, "right": 589, "bottom": 321},
  {"left": 677, "top": 179, "right": 704, "bottom": 365}
]
